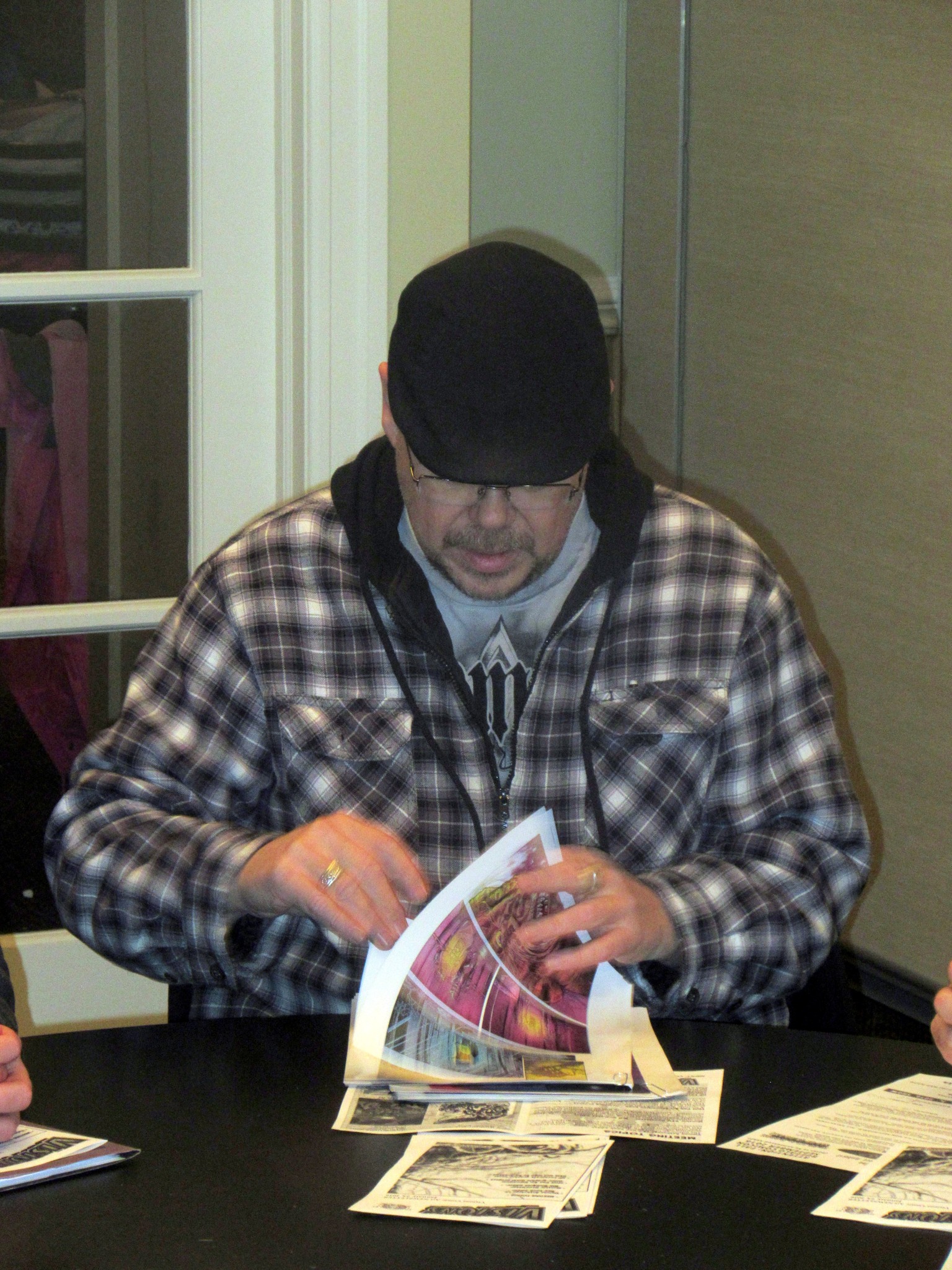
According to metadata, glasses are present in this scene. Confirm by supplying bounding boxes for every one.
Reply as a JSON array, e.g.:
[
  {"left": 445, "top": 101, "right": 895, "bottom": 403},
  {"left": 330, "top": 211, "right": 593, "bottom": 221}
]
[{"left": 404, "top": 434, "right": 588, "bottom": 513}]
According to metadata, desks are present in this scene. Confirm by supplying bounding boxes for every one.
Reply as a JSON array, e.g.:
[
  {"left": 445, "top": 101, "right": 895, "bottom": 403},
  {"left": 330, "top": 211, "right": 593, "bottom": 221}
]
[{"left": 0, "top": 1012, "right": 952, "bottom": 1270}]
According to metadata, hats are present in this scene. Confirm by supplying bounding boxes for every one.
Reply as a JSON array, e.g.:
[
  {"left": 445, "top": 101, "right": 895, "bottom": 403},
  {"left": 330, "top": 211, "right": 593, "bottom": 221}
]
[{"left": 387, "top": 240, "right": 612, "bottom": 487}]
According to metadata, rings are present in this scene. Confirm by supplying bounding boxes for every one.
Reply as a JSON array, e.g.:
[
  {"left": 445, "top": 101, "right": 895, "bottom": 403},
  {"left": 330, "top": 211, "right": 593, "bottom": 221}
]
[
  {"left": 578, "top": 860, "right": 602, "bottom": 901},
  {"left": 318, "top": 859, "right": 343, "bottom": 890}
]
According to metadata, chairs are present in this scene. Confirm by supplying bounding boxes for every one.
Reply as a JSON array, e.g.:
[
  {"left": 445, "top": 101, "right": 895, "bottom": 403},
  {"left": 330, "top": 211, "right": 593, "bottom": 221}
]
[{"left": 0, "top": 927, "right": 170, "bottom": 1040}]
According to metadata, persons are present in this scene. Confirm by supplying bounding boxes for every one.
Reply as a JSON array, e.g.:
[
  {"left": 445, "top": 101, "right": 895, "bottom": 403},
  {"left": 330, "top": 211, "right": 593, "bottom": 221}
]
[
  {"left": 930, "top": 959, "right": 952, "bottom": 1069},
  {"left": 44, "top": 240, "right": 870, "bottom": 1029},
  {"left": 0, "top": 1023, "right": 32, "bottom": 1142}
]
